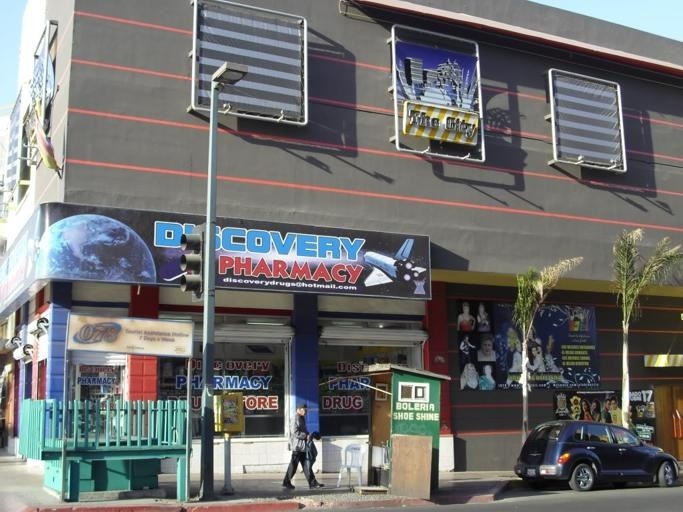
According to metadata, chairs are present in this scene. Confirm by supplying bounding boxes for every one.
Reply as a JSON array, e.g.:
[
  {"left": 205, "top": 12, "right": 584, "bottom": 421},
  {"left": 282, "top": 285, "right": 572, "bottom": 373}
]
[{"left": 335, "top": 442, "right": 365, "bottom": 494}]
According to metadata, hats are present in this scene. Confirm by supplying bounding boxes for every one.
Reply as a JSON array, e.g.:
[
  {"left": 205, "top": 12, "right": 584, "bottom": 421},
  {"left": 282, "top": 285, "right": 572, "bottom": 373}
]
[{"left": 297, "top": 404, "right": 307, "bottom": 408}]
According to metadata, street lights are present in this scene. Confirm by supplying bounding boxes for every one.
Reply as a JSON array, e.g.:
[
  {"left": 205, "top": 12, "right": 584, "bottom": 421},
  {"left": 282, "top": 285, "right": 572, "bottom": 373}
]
[{"left": 193, "top": 60, "right": 251, "bottom": 501}]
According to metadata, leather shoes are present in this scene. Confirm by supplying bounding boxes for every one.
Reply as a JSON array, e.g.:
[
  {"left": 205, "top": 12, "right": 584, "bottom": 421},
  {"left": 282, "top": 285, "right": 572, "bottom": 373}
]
[
  {"left": 283, "top": 482, "right": 295, "bottom": 489},
  {"left": 310, "top": 484, "right": 324, "bottom": 488}
]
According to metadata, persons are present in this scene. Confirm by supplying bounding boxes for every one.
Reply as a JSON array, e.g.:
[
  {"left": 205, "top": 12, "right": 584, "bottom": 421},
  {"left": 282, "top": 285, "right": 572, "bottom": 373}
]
[
  {"left": 580, "top": 396, "right": 622, "bottom": 425},
  {"left": 283, "top": 404, "right": 325, "bottom": 489},
  {"left": 457, "top": 301, "right": 558, "bottom": 392}
]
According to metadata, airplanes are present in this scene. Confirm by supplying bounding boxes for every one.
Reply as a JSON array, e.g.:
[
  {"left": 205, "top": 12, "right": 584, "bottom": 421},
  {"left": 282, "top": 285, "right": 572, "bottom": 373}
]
[{"left": 361, "top": 237, "right": 427, "bottom": 291}]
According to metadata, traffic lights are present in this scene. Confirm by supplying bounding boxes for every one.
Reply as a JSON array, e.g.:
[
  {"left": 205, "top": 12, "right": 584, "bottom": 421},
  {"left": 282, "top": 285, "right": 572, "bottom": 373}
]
[{"left": 179, "top": 220, "right": 203, "bottom": 296}]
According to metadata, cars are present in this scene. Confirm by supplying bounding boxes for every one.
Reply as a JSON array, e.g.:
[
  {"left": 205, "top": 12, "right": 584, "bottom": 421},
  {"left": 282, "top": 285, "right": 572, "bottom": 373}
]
[{"left": 508, "top": 419, "right": 680, "bottom": 493}]
